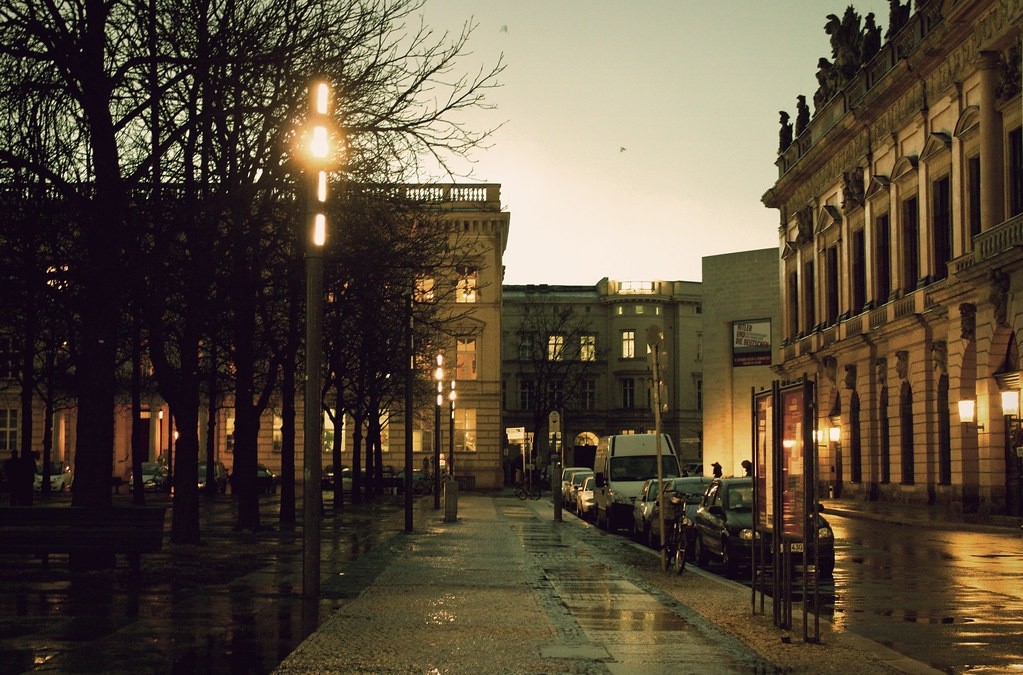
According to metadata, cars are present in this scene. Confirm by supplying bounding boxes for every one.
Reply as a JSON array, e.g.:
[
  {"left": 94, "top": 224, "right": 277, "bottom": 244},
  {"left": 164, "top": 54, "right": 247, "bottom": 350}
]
[
  {"left": 693, "top": 476, "right": 834, "bottom": 578},
  {"left": 230, "top": 464, "right": 277, "bottom": 496},
  {"left": 198, "top": 461, "right": 227, "bottom": 495},
  {"left": 681, "top": 462, "right": 703, "bottom": 477},
  {"left": 128, "top": 462, "right": 174, "bottom": 495},
  {"left": 323, "top": 464, "right": 433, "bottom": 499},
  {"left": 0, "top": 458, "right": 11, "bottom": 491},
  {"left": 561, "top": 468, "right": 593, "bottom": 509},
  {"left": 33, "top": 461, "right": 73, "bottom": 493},
  {"left": 574, "top": 477, "right": 594, "bottom": 521},
  {"left": 648, "top": 478, "right": 741, "bottom": 552},
  {"left": 633, "top": 479, "right": 674, "bottom": 545}
]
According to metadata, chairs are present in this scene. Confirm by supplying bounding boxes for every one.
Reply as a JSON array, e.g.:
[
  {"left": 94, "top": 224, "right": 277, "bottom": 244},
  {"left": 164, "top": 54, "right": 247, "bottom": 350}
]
[
  {"left": 727, "top": 491, "right": 744, "bottom": 507},
  {"left": 612, "top": 467, "right": 628, "bottom": 479}
]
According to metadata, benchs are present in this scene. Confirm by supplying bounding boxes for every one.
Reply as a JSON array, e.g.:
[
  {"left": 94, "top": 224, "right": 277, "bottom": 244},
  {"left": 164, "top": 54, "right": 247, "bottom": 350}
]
[
  {"left": 256, "top": 477, "right": 273, "bottom": 494},
  {"left": 111, "top": 477, "right": 122, "bottom": 494},
  {"left": 372, "top": 477, "right": 405, "bottom": 495}
]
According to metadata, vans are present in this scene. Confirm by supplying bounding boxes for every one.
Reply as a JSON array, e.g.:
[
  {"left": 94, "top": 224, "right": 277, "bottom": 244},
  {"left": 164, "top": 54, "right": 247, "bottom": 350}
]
[{"left": 593, "top": 433, "right": 683, "bottom": 534}]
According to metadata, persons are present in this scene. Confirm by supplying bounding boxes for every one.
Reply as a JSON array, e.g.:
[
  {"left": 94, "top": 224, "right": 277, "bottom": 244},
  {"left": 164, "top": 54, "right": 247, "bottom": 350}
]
[
  {"left": 681, "top": 468, "right": 688, "bottom": 478},
  {"left": 741, "top": 460, "right": 753, "bottom": 477},
  {"left": 711, "top": 463, "right": 722, "bottom": 479}
]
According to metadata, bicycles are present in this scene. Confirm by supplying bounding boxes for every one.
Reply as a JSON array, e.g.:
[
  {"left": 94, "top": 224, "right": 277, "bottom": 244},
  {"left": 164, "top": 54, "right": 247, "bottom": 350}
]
[
  {"left": 513, "top": 481, "right": 541, "bottom": 500},
  {"left": 663, "top": 489, "right": 709, "bottom": 574}
]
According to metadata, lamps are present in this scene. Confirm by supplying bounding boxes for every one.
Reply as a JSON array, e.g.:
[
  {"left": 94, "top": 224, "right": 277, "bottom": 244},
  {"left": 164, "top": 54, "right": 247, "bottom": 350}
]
[
  {"left": 1000, "top": 386, "right": 1023, "bottom": 427},
  {"left": 958, "top": 397, "right": 984, "bottom": 433},
  {"left": 812, "top": 425, "right": 841, "bottom": 447}
]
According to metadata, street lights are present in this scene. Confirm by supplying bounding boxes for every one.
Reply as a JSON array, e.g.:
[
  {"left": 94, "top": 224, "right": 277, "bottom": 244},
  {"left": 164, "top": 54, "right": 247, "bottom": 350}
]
[
  {"left": 405, "top": 292, "right": 414, "bottom": 532},
  {"left": 449, "top": 380, "right": 455, "bottom": 475},
  {"left": 159, "top": 409, "right": 163, "bottom": 455},
  {"left": 434, "top": 354, "right": 443, "bottom": 511},
  {"left": 305, "top": 80, "right": 330, "bottom": 636}
]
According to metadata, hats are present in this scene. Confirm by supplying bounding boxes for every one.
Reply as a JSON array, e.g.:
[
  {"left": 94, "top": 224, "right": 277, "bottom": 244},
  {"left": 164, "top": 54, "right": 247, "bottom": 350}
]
[{"left": 711, "top": 462, "right": 722, "bottom": 469}]
